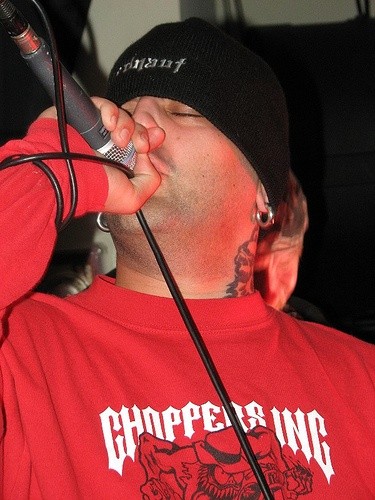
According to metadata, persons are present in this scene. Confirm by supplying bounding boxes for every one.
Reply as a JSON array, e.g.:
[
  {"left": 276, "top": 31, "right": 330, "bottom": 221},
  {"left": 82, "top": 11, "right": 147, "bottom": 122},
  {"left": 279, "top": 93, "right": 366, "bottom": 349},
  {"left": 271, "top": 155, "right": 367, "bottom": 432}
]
[{"left": 0, "top": 20, "right": 375, "bottom": 500}]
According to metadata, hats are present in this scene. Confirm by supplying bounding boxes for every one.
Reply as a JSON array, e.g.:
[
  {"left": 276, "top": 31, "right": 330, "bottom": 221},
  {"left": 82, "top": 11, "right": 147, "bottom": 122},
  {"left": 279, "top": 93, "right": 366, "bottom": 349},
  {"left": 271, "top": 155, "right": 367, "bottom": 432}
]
[{"left": 106, "top": 15, "right": 292, "bottom": 217}]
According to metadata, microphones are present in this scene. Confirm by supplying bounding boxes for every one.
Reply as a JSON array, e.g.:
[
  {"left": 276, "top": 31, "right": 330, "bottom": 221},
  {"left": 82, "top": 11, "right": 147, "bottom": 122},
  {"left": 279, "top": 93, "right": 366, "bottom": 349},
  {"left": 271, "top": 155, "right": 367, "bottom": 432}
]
[{"left": 0, "top": 0, "right": 138, "bottom": 176}]
